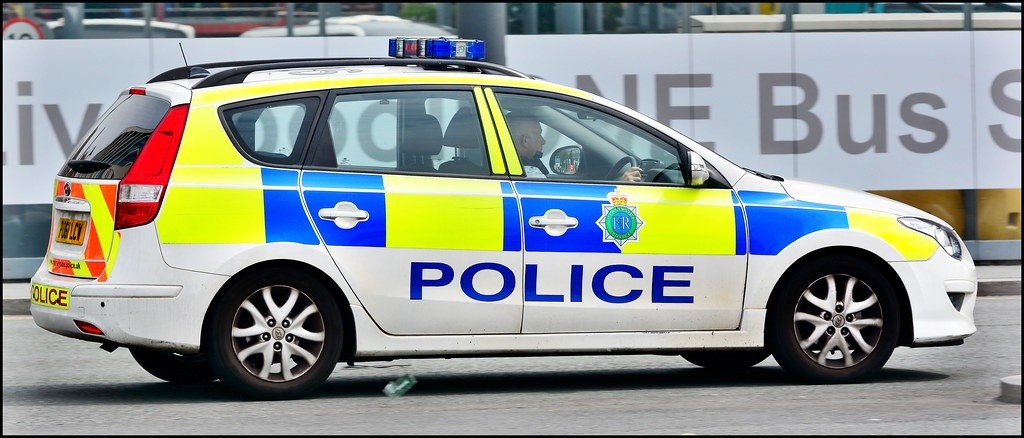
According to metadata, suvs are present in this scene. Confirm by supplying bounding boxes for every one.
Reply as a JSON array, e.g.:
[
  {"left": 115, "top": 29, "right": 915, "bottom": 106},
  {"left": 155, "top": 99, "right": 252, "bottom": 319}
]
[{"left": 47, "top": 14, "right": 459, "bottom": 39}]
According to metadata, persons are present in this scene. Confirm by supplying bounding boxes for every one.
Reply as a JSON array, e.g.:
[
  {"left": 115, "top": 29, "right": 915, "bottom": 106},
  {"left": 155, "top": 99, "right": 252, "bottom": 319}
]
[{"left": 506, "top": 109, "right": 642, "bottom": 184}]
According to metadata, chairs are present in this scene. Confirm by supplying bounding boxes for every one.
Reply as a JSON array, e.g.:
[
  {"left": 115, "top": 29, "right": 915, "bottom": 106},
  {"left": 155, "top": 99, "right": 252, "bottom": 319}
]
[
  {"left": 437, "top": 107, "right": 489, "bottom": 175},
  {"left": 401, "top": 113, "right": 443, "bottom": 173}
]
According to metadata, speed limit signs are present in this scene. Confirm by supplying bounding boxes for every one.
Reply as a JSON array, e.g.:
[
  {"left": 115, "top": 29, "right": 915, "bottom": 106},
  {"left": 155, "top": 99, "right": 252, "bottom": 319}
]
[{"left": 2, "top": 19, "right": 38, "bottom": 40}]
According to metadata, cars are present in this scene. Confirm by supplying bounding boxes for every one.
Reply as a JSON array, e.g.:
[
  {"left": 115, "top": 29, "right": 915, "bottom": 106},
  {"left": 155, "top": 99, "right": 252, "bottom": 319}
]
[{"left": 29, "top": 37, "right": 980, "bottom": 400}]
